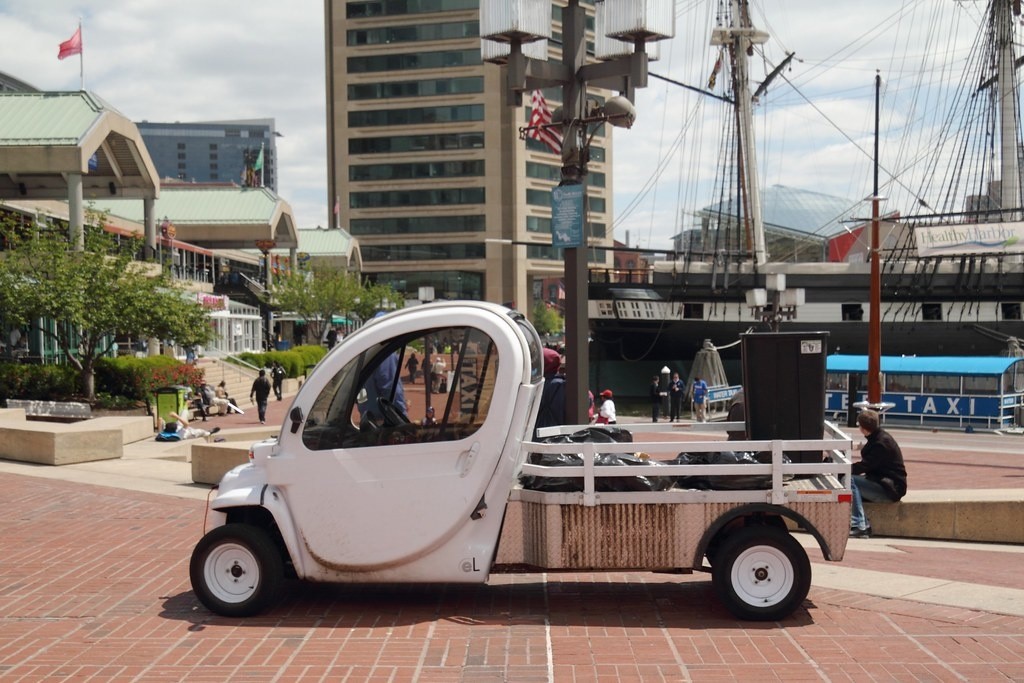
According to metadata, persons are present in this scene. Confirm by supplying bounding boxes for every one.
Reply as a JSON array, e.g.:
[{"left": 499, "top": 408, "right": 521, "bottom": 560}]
[
  {"left": 598, "top": 390, "right": 616, "bottom": 424},
  {"left": 405, "top": 353, "right": 419, "bottom": 383},
  {"left": 841, "top": 409, "right": 907, "bottom": 539},
  {"left": 326, "top": 328, "right": 343, "bottom": 349},
  {"left": 650, "top": 376, "right": 667, "bottom": 423},
  {"left": 250, "top": 370, "right": 272, "bottom": 424},
  {"left": 11, "top": 333, "right": 33, "bottom": 364},
  {"left": 357, "top": 351, "right": 408, "bottom": 426},
  {"left": 690, "top": 377, "right": 708, "bottom": 422},
  {"left": 187, "top": 382, "right": 210, "bottom": 421},
  {"left": 726, "top": 401, "right": 746, "bottom": 441},
  {"left": 270, "top": 361, "right": 286, "bottom": 401},
  {"left": 158, "top": 412, "right": 221, "bottom": 439},
  {"left": 431, "top": 357, "right": 446, "bottom": 394},
  {"left": 669, "top": 373, "right": 684, "bottom": 422},
  {"left": 215, "top": 381, "right": 239, "bottom": 413},
  {"left": 422, "top": 407, "right": 437, "bottom": 425}
]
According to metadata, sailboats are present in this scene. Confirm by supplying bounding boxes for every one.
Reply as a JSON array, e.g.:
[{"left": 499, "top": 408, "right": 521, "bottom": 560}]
[{"left": 585, "top": 0, "right": 1024, "bottom": 398}]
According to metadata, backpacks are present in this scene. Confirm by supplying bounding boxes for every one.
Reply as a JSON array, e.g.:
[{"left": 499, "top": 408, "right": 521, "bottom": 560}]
[
  {"left": 273, "top": 366, "right": 283, "bottom": 380},
  {"left": 156, "top": 430, "right": 180, "bottom": 440},
  {"left": 533, "top": 378, "right": 563, "bottom": 441}
]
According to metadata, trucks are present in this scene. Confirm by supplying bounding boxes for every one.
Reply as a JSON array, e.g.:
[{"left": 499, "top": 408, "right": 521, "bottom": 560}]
[{"left": 189, "top": 302, "right": 855, "bottom": 616}]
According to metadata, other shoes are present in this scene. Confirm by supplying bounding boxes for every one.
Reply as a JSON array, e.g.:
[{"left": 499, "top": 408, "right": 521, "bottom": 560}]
[{"left": 210, "top": 427, "right": 220, "bottom": 434}]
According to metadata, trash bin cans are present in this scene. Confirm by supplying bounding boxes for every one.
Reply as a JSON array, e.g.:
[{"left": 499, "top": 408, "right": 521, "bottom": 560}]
[
  {"left": 276, "top": 341, "right": 289, "bottom": 351},
  {"left": 157, "top": 385, "right": 190, "bottom": 430}
]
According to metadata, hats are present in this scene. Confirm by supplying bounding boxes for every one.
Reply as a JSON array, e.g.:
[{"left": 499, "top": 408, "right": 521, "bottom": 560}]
[
  {"left": 544, "top": 347, "right": 561, "bottom": 372},
  {"left": 599, "top": 389, "right": 612, "bottom": 398},
  {"left": 427, "top": 407, "right": 434, "bottom": 413}
]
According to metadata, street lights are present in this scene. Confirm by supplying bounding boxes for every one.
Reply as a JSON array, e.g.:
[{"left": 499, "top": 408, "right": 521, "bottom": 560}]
[{"left": 480, "top": 6, "right": 673, "bottom": 425}]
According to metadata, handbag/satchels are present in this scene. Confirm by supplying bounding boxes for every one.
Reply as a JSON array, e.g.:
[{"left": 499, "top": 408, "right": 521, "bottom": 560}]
[
  {"left": 596, "top": 412, "right": 608, "bottom": 424},
  {"left": 430, "top": 372, "right": 436, "bottom": 381}
]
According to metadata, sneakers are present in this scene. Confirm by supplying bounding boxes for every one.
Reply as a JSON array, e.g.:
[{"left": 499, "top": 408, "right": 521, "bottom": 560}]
[{"left": 848, "top": 524, "right": 873, "bottom": 539}]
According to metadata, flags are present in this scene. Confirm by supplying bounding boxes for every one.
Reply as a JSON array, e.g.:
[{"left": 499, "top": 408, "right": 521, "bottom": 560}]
[
  {"left": 527, "top": 90, "right": 563, "bottom": 155},
  {"left": 58, "top": 29, "right": 81, "bottom": 61},
  {"left": 708, "top": 71, "right": 716, "bottom": 89},
  {"left": 255, "top": 149, "right": 262, "bottom": 171}
]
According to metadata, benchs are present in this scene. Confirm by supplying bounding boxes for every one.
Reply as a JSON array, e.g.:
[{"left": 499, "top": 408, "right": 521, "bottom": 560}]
[
  {"left": 5, "top": 398, "right": 92, "bottom": 420},
  {"left": 190, "top": 389, "right": 229, "bottom": 420}
]
